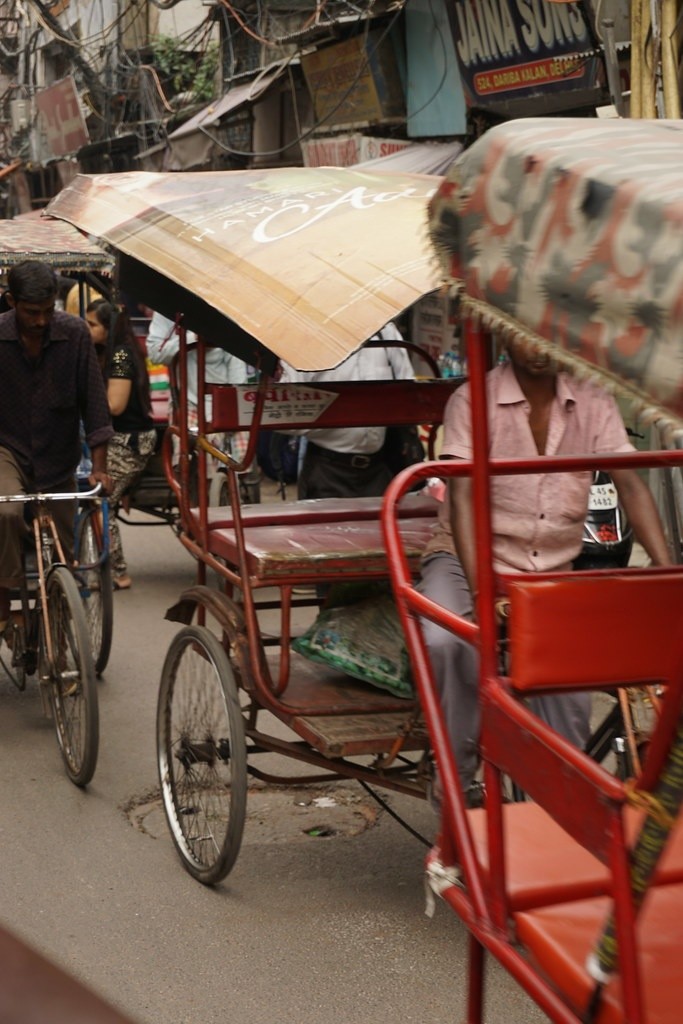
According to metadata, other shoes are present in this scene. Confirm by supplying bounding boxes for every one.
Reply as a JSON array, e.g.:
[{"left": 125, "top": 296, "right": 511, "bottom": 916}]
[{"left": 427, "top": 779, "right": 486, "bottom": 814}]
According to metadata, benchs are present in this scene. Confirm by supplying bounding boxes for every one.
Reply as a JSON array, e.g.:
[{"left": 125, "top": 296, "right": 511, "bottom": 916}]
[
  {"left": 127, "top": 317, "right": 172, "bottom": 425},
  {"left": 454, "top": 564, "right": 683, "bottom": 1023},
  {"left": 174, "top": 371, "right": 472, "bottom": 580}
]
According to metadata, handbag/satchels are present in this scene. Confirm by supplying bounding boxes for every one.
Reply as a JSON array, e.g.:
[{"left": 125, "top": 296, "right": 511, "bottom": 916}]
[{"left": 387, "top": 425, "right": 426, "bottom": 493}]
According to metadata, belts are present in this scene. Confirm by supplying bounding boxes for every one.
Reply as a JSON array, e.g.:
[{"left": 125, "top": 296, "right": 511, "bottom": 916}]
[{"left": 300, "top": 438, "right": 387, "bottom": 469}]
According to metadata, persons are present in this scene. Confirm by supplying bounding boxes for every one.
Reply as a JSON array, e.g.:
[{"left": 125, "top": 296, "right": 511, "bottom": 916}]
[
  {"left": 411, "top": 332, "right": 673, "bottom": 820},
  {"left": 0, "top": 259, "right": 117, "bottom": 698},
  {"left": 83, "top": 298, "right": 158, "bottom": 591},
  {"left": 145, "top": 310, "right": 262, "bottom": 508},
  {"left": 275, "top": 321, "right": 428, "bottom": 611}
]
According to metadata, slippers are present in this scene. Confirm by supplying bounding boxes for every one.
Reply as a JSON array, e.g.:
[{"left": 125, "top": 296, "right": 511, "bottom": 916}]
[
  {"left": 92, "top": 581, "right": 130, "bottom": 591},
  {"left": 0, "top": 602, "right": 11, "bottom": 645},
  {"left": 63, "top": 681, "right": 77, "bottom": 697}
]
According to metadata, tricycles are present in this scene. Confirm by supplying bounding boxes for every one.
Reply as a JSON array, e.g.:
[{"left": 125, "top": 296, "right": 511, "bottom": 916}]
[
  {"left": 1, "top": 218, "right": 268, "bottom": 787},
  {"left": 40, "top": 166, "right": 653, "bottom": 887},
  {"left": 382, "top": 117, "right": 682, "bottom": 1024}
]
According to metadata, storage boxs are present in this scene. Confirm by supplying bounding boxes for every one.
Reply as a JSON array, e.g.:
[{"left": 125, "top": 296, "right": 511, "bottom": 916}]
[{"left": 300, "top": 26, "right": 408, "bottom": 125}]
